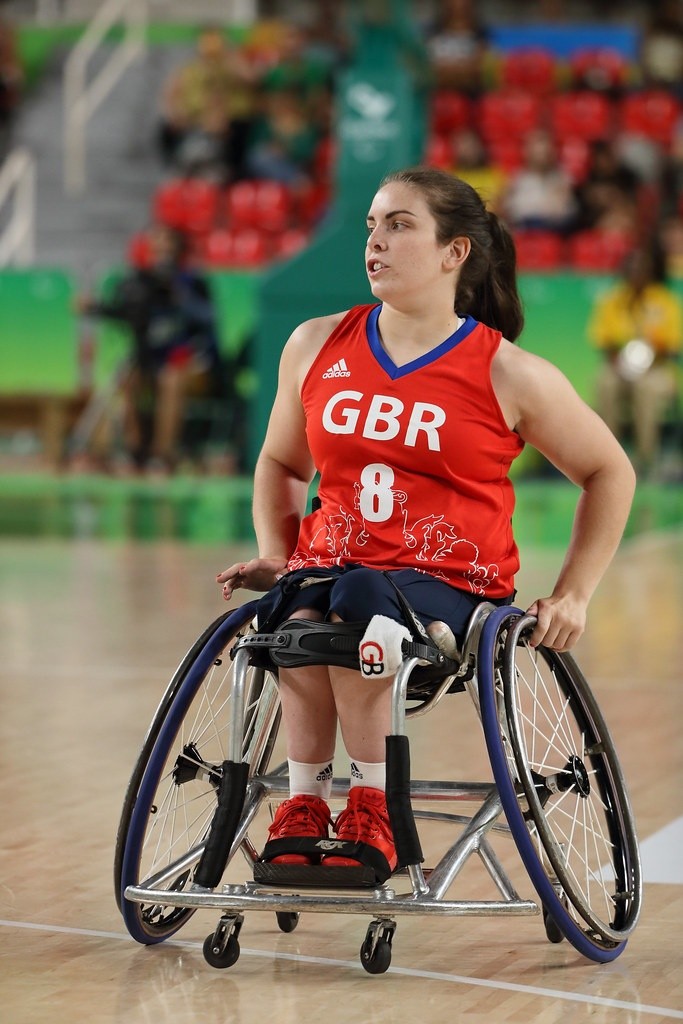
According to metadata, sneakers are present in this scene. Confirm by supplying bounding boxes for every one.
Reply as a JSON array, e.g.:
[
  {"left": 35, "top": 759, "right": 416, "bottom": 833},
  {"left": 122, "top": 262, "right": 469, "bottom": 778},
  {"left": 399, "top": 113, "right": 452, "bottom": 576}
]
[
  {"left": 322, "top": 785, "right": 399, "bottom": 873},
  {"left": 265, "top": 794, "right": 335, "bottom": 866}
]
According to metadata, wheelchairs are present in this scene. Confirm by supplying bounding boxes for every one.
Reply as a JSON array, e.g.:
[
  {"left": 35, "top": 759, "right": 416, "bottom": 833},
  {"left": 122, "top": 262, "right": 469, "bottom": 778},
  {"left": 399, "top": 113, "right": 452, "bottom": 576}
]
[{"left": 114, "top": 497, "right": 642, "bottom": 981}]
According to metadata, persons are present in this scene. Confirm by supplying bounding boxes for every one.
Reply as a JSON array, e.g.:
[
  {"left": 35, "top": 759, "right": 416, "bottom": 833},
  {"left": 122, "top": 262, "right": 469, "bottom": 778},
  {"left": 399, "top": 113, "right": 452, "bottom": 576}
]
[
  {"left": 214, "top": 166, "right": 640, "bottom": 889},
  {"left": 71, "top": 0, "right": 683, "bottom": 480}
]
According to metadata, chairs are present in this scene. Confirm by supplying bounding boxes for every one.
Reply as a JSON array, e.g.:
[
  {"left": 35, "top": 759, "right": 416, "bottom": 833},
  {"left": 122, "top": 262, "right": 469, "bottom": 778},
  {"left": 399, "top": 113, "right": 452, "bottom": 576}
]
[
  {"left": 429, "top": 46, "right": 682, "bottom": 424},
  {"left": 1, "top": 176, "right": 309, "bottom": 476}
]
